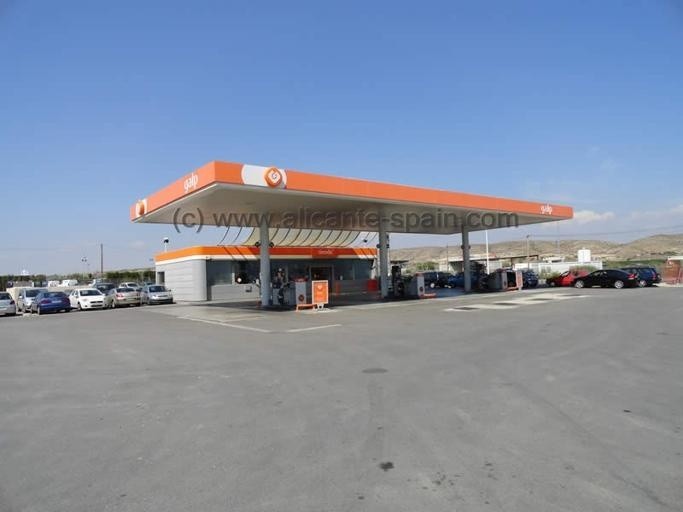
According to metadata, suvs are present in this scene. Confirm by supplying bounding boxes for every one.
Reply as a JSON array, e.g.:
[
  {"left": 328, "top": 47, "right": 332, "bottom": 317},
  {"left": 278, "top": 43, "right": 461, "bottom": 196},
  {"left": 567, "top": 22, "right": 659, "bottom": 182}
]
[{"left": 621, "top": 267, "right": 662, "bottom": 287}]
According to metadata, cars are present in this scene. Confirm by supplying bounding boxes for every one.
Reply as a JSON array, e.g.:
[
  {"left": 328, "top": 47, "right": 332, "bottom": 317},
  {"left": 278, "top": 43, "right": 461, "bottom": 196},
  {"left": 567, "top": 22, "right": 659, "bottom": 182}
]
[
  {"left": 0, "top": 279, "right": 176, "bottom": 315},
  {"left": 545, "top": 268, "right": 591, "bottom": 288},
  {"left": 388, "top": 268, "right": 539, "bottom": 290},
  {"left": 570, "top": 268, "right": 639, "bottom": 288}
]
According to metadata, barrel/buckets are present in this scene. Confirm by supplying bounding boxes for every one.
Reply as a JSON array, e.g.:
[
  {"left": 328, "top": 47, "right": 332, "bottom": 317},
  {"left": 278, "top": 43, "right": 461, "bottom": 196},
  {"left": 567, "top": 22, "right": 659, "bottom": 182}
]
[{"left": 367, "top": 280, "right": 378, "bottom": 292}]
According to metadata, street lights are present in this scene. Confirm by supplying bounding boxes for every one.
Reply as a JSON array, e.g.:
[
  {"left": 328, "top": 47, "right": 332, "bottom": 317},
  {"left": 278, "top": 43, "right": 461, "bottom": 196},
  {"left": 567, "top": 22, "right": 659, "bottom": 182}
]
[
  {"left": 80, "top": 255, "right": 88, "bottom": 280},
  {"left": 526, "top": 234, "right": 530, "bottom": 268}
]
